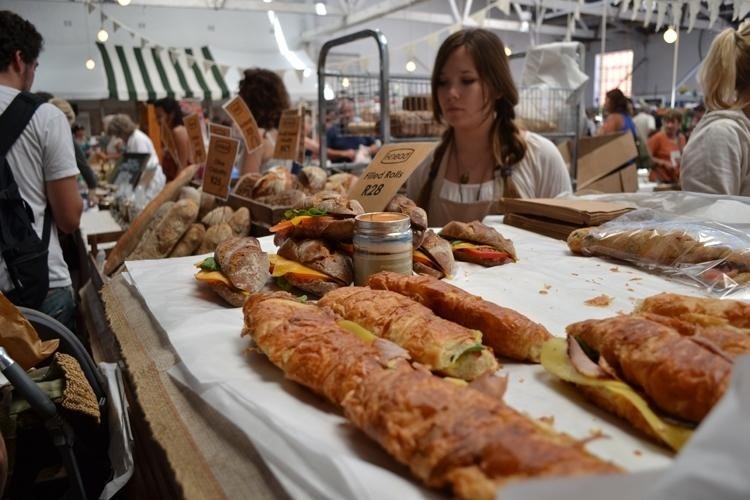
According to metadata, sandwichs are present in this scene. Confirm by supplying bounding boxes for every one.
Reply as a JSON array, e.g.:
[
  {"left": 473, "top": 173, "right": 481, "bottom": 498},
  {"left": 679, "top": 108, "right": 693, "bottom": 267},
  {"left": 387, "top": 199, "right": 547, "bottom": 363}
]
[{"left": 193, "top": 193, "right": 518, "bottom": 308}]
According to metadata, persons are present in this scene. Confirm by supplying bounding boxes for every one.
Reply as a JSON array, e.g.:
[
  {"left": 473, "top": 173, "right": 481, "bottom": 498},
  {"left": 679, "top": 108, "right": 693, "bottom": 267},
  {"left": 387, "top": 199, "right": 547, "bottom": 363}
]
[
  {"left": 299, "top": 89, "right": 399, "bottom": 173},
  {"left": 407, "top": 28, "right": 573, "bottom": 228},
  {"left": 1, "top": 11, "right": 84, "bottom": 353},
  {"left": 39, "top": 92, "right": 231, "bottom": 208},
  {"left": 579, "top": 88, "right": 707, "bottom": 183},
  {"left": 679, "top": 19, "right": 750, "bottom": 197},
  {"left": 237, "top": 67, "right": 290, "bottom": 178}
]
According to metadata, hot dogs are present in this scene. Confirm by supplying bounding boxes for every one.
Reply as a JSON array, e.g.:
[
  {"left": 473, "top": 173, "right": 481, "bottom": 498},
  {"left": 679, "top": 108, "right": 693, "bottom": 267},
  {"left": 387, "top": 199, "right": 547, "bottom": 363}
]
[
  {"left": 315, "top": 284, "right": 499, "bottom": 380},
  {"left": 340, "top": 358, "right": 627, "bottom": 498},
  {"left": 369, "top": 269, "right": 551, "bottom": 364},
  {"left": 540, "top": 292, "right": 748, "bottom": 454},
  {"left": 240, "top": 290, "right": 390, "bottom": 409}
]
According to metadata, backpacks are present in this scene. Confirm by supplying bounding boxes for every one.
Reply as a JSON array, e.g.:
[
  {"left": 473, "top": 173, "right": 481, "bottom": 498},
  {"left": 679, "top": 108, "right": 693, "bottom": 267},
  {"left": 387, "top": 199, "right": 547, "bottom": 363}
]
[{"left": 0, "top": 90, "right": 52, "bottom": 306}]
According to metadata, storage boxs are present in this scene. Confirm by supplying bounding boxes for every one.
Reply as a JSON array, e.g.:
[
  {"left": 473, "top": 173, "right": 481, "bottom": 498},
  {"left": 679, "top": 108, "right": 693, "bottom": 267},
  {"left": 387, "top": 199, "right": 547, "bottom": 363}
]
[
  {"left": 222, "top": 177, "right": 296, "bottom": 238},
  {"left": 554, "top": 126, "right": 639, "bottom": 191},
  {"left": 573, "top": 163, "right": 641, "bottom": 194}
]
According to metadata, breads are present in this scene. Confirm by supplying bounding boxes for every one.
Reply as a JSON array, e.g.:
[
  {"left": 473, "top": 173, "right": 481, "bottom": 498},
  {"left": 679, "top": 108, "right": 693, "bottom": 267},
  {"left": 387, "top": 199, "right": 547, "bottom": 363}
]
[{"left": 128, "top": 162, "right": 356, "bottom": 260}]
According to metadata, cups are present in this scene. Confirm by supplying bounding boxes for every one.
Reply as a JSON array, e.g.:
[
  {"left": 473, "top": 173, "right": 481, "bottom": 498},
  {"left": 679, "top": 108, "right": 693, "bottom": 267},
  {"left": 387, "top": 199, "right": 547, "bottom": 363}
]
[{"left": 352, "top": 212, "right": 414, "bottom": 287}]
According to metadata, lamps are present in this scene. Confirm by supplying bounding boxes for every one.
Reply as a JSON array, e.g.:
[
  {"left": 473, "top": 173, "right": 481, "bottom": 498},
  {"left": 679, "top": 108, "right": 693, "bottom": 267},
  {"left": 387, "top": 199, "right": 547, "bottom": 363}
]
[
  {"left": 503, "top": 45, "right": 515, "bottom": 58},
  {"left": 406, "top": 54, "right": 417, "bottom": 74},
  {"left": 312, "top": 1, "right": 330, "bottom": 20},
  {"left": 662, "top": 14, "right": 679, "bottom": 45},
  {"left": 342, "top": 76, "right": 352, "bottom": 88},
  {"left": 87, "top": 1, "right": 130, "bottom": 72}
]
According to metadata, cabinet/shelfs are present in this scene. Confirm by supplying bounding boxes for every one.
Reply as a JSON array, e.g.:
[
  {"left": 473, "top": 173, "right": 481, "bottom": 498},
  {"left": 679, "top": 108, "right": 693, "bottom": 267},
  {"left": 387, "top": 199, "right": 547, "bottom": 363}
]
[{"left": 316, "top": 27, "right": 588, "bottom": 196}]
